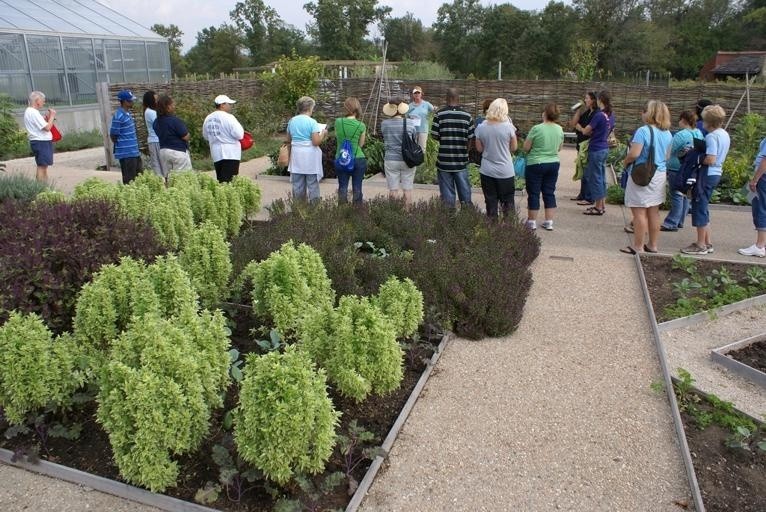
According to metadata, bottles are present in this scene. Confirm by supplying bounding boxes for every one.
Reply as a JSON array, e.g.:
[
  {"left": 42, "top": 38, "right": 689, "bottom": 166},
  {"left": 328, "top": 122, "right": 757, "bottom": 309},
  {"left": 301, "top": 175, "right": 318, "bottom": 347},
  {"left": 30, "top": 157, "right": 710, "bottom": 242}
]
[{"left": 686, "top": 142, "right": 691, "bottom": 152}]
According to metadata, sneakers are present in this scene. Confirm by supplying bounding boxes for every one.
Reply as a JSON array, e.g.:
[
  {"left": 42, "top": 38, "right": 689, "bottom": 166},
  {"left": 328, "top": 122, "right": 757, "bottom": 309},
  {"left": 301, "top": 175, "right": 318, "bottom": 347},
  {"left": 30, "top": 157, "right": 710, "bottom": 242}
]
[
  {"left": 619, "top": 224, "right": 713, "bottom": 255},
  {"left": 523, "top": 220, "right": 536, "bottom": 230},
  {"left": 738, "top": 244, "right": 765, "bottom": 257},
  {"left": 542, "top": 221, "right": 553, "bottom": 229}
]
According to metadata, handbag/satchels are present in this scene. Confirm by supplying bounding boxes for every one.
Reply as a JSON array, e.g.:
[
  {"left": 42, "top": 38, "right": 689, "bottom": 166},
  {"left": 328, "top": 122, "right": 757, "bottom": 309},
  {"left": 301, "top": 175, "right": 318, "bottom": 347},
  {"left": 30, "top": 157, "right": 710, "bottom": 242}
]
[
  {"left": 277, "top": 145, "right": 288, "bottom": 171},
  {"left": 607, "top": 128, "right": 617, "bottom": 147},
  {"left": 402, "top": 119, "right": 424, "bottom": 168},
  {"left": 44, "top": 116, "right": 62, "bottom": 141},
  {"left": 631, "top": 145, "right": 658, "bottom": 186}
]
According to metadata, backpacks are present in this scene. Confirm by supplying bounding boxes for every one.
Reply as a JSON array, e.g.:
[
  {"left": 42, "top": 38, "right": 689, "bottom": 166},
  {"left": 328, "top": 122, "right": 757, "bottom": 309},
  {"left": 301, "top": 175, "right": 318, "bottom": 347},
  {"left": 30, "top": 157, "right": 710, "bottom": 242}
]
[{"left": 335, "top": 118, "right": 362, "bottom": 173}]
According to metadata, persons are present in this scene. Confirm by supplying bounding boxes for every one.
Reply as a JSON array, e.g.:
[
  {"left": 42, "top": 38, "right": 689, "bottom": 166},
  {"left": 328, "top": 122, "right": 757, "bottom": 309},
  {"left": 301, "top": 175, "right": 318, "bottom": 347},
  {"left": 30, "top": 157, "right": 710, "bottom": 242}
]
[
  {"left": 110, "top": 90, "right": 143, "bottom": 189},
  {"left": 571, "top": 90, "right": 616, "bottom": 216},
  {"left": 286, "top": 97, "right": 329, "bottom": 202},
  {"left": 619, "top": 99, "right": 730, "bottom": 255},
  {"left": 380, "top": 86, "right": 564, "bottom": 231},
  {"left": 739, "top": 137, "right": 766, "bottom": 257},
  {"left": 334, "top": 97, "right": 367, "bottom": 206},
  {"left": 24, "top": 91, "right": 56, "bottom": 185},
  {"left": 202, "top": 95, "right": 244, "bottom": 182},
  {"left": 143, "top": 91, "right": 193, "bottom": 179}
]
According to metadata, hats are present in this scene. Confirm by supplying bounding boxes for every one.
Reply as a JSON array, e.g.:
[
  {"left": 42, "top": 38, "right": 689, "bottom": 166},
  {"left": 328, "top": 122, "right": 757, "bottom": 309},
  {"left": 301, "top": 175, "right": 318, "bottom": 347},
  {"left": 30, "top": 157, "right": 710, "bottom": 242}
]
[
  {"left": 215, "top": 95, "right": 236, "bottom": 104},
  {"left": 413, "top": 86, "right": 421, "bottom": 93},
  {"left": 118, "top": 92, "right": 137, "bottom": 101}
]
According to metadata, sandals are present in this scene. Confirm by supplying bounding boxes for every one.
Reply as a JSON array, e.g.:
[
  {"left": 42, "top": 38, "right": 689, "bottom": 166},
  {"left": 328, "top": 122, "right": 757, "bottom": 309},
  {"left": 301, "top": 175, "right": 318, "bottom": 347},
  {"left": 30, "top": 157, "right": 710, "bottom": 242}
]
[{"left": 571, "top": 198, "right": 606, "bottom": 215}]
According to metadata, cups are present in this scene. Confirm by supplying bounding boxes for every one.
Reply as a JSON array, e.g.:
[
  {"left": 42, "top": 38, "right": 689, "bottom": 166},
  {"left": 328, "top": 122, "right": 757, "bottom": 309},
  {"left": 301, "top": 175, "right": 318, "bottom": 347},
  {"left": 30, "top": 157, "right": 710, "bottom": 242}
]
[{"left": 570, "top": 101, "right": 584, "bottom": 113}]
